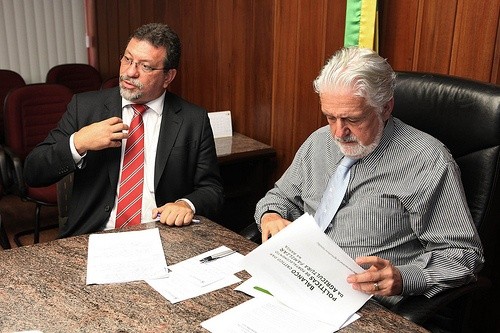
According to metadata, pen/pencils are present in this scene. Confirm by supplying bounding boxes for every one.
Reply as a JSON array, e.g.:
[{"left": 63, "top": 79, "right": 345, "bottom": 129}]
[
  {"left": 157, "top": 213, "right": 200, "bottom": 223},
  {"left": 199, "top": 247, "right": 240, "bottom": 263}
]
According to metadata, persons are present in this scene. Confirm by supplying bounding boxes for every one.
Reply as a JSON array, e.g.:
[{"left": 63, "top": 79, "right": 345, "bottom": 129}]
[
  {"left": 20, "top": 22, "right": 222, "bottom": 240},
  {"left": 254, "top": 46, "right": 485, "bottom": 311}
]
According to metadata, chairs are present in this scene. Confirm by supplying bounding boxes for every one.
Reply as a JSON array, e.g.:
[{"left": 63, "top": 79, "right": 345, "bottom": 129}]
[
  {"left": 391, "top": 72, "right": 500, "bottom": 333},
  {"left": 0, "top": 62, "right": 106, "bottom": 251}
]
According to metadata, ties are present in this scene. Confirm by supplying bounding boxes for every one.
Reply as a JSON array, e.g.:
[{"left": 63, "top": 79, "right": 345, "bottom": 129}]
[
  {"left": 116, "top": 104, "right": 149, "bottom": 228},
  {"left": 313, "top": 156, "right": 359, "bottom": 232}
]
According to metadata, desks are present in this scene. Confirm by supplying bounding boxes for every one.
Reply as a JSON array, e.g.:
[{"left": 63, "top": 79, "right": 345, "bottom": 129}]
[
  {"left": 0, "top": 213, "right": 430, "bottom": 333},
  {"left": 207, "top": 131, "right": 278, "bottom": 224}
]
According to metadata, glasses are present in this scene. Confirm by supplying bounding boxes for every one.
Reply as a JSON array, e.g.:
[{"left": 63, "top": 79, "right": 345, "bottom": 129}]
[{"left": 119, "top": 53, "right": 169, "bottom": 73}]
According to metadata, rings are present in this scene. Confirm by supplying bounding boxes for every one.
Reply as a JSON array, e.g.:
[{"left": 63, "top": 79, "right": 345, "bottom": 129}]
[{"left": 374, "top": 281, "right": 380, "bottom": 290}]
